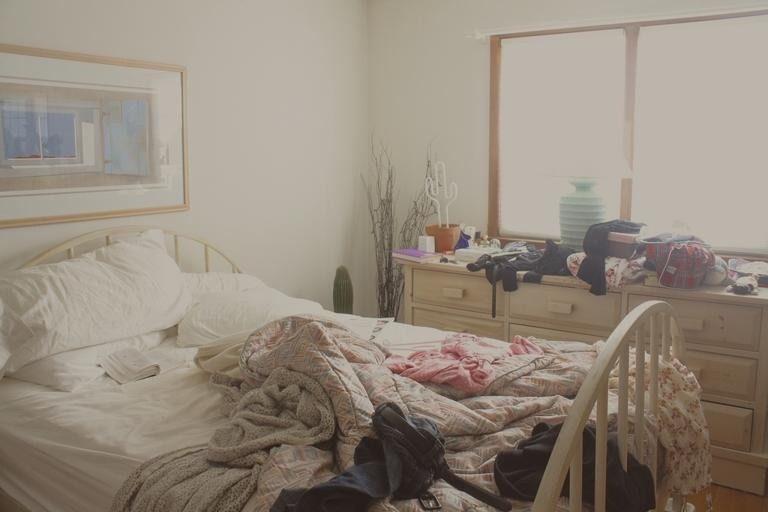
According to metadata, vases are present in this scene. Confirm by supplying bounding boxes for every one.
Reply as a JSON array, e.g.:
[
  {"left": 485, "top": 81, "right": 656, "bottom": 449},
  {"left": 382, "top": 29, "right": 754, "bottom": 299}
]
[
  {"left": 426, "top": 223, "right": 462, "bottom": 252},
  {"left": 560, "top": 174, "right": 607, "bottom": 252}
]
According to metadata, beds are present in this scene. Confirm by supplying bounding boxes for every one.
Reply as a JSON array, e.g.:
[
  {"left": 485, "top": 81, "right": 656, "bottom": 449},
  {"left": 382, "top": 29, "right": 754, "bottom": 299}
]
[{"left": 0, "top": 225, "right": 688, "bottom": 511}]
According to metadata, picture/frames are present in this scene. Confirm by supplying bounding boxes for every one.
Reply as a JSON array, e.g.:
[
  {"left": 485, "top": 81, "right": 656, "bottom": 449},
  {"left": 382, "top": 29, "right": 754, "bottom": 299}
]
[{"left": 0, "top": 42, "right": 190, "bottom": 226}]
